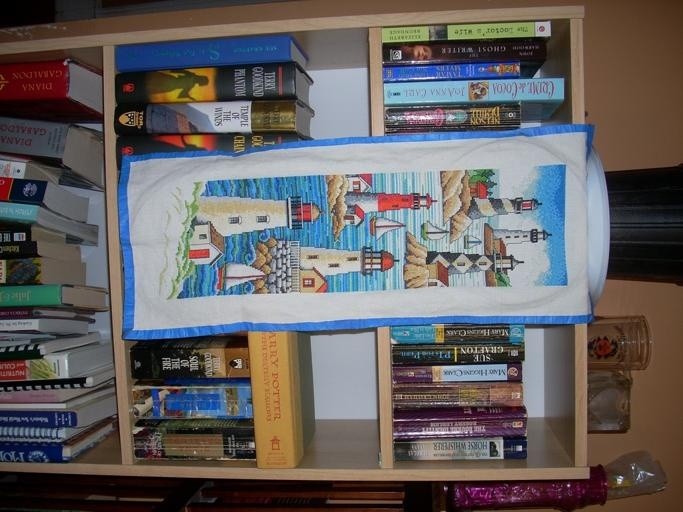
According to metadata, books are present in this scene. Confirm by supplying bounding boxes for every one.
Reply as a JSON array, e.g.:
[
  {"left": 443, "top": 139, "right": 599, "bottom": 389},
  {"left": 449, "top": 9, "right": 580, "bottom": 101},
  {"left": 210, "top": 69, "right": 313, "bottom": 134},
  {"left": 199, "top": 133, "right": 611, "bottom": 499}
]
[
  {"left": 390, "top": 324, "right": 527, "bottom": 462},
  {"left": 113, "top": 37, "right": 315, "bottom": 182},
  {"left": 382, "top": 20, "right": 565, "bottom": 135},
  {"left": 129, "top": 334, "right": 257, "bottom": 459},
  {"left": 1, "top": 57, "right": 118, "bottom": 464}
]
